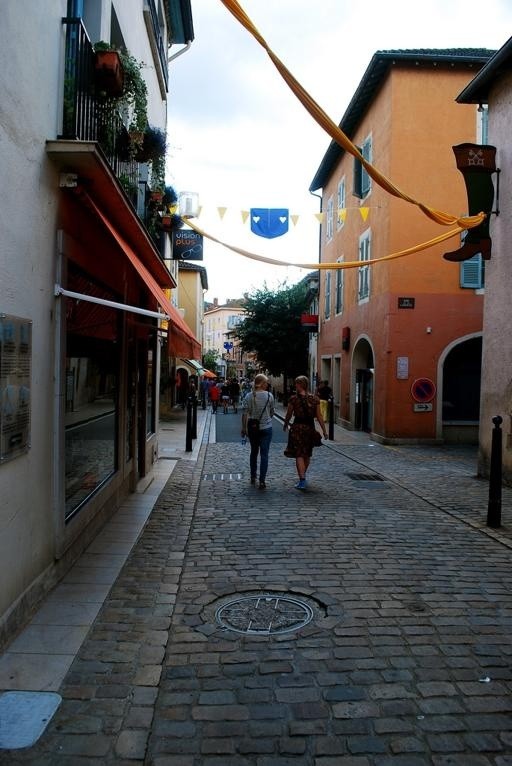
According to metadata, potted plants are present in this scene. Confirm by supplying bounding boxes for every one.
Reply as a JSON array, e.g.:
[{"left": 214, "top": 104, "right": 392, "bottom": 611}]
[
  {"left": 96, "top": 41, "right": 147, "bottom": 142},
  {"left": 153, "top": 183, "right": 182, "bottom": 230}
]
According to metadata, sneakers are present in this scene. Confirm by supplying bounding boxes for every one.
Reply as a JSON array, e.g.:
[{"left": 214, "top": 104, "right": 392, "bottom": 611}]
[
  {"left": 295, "top": 480, "right": 306, "bottom": 489},
  {"left": 251, "top": 475, "right": 266, "bottom": 488}
]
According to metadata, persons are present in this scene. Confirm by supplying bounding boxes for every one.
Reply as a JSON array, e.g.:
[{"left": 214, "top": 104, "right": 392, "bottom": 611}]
[
  {"left": 239, "top": 373, "right": 274, "bottom": 490},
  {"left": 282, "top": 374, "right": 328, "bottom": 490},
  {"left": 199, "top": 370, "right": 257, "bottom": 415},
  {"left": 313, "top": 380, "right": 324, "bottom": 421},
  {"left": 317, "top": 380, "right": 332, "bottom": 423}
]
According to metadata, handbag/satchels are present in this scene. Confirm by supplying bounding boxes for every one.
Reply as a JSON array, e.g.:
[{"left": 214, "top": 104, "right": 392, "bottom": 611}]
[
  {"left": 309, "top": 430, "right": 323, "bottom": 447},
  {"left": 247, "top": 417, "right": 261, "bottom": 445}
]
